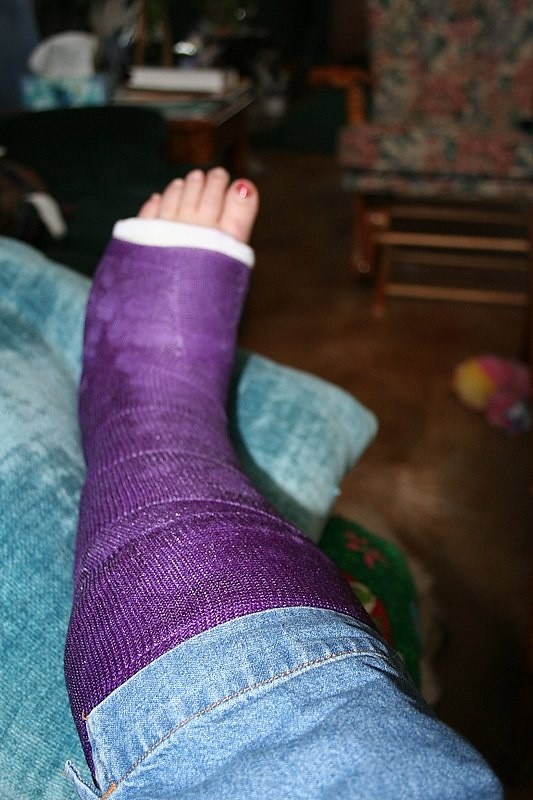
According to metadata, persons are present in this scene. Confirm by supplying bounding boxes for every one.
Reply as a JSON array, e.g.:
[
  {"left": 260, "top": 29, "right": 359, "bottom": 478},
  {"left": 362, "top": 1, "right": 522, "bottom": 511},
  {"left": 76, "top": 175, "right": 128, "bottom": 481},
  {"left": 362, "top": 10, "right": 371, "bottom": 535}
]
[{"left": 60, "top": 166, "right": 506, "bottom": 800}]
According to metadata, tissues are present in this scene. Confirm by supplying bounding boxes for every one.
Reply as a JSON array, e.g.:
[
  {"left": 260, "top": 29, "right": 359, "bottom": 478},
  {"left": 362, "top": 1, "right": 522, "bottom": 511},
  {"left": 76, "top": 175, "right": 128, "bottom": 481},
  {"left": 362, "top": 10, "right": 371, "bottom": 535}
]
[{"left": 17, "top": 31, "right": 110, "bottom": 113}]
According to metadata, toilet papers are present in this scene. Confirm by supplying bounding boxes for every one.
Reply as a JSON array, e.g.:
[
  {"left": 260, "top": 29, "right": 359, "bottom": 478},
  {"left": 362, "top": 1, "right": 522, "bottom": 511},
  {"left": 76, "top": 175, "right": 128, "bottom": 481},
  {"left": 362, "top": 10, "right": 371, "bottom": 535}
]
[{"left": 128, "top": 64, "right": 227, "bottom": 93}]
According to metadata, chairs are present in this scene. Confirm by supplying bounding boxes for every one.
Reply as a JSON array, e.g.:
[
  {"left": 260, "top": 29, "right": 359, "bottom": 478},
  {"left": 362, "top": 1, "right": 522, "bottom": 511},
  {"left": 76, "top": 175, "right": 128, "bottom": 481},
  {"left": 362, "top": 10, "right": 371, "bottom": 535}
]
[{"left": 327, "top": 0, "right": 533, "bottom": 311}]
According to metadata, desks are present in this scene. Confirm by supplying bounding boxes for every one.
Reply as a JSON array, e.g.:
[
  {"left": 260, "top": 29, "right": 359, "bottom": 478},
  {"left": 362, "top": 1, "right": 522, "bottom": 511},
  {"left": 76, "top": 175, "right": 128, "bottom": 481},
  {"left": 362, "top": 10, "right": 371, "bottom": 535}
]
[{"left": 111, "top": 79, "right": 256, "bottom": 164}]
